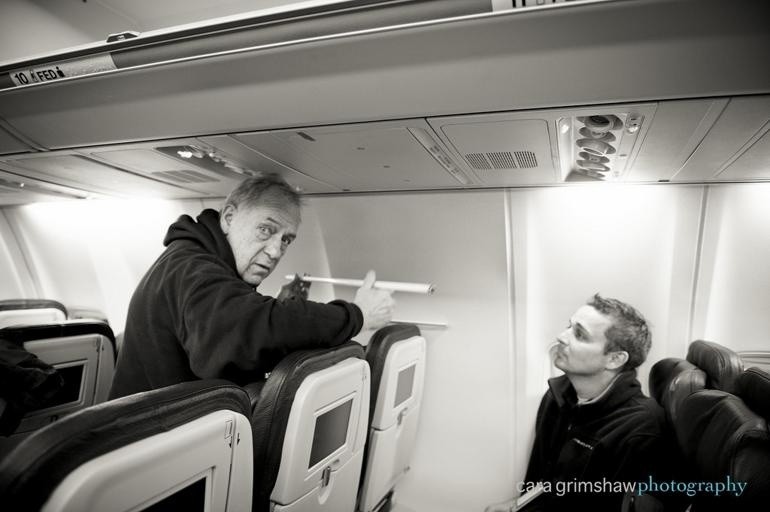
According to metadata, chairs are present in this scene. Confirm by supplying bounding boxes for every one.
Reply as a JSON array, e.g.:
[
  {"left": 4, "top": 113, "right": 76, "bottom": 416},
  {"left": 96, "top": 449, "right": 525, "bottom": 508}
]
[
  {"left": 686, "top": 339, "right": 744, "bottom": 395},
  {"left": 649, "top": 353, "right": 707, "bottom": 434},
  {"left": 0, "top": 298, "right": 427, "bottom": 509},
  {"left": 730, "top": 362, "right": 770, "bottom": 417},
  {"left": 671, "top": 387, "right": 770, "bottom": 512}
]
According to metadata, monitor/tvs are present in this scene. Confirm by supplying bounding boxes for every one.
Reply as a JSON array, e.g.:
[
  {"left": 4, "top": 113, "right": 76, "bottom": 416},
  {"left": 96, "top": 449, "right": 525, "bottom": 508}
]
[
  {"left": 306, "top": 393, "right": 353, "bottom": 474},
  {"left": 394, "top": 361, "right": 415, "bottom": 411},
  {"left": 32, "top": 362, "right": 87, "bottom": 412},
  {"left": 123, "top": 469, "right": 212, "bottom": 512}
]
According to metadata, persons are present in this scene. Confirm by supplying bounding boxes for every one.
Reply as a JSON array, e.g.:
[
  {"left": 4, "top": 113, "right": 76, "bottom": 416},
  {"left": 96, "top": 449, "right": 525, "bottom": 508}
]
[
  {"left": 107, "top": 172, "right": 395, "bottom": 401},
  {"left": 487, "top": 293, "right": 667, "bottom": 512}
]
